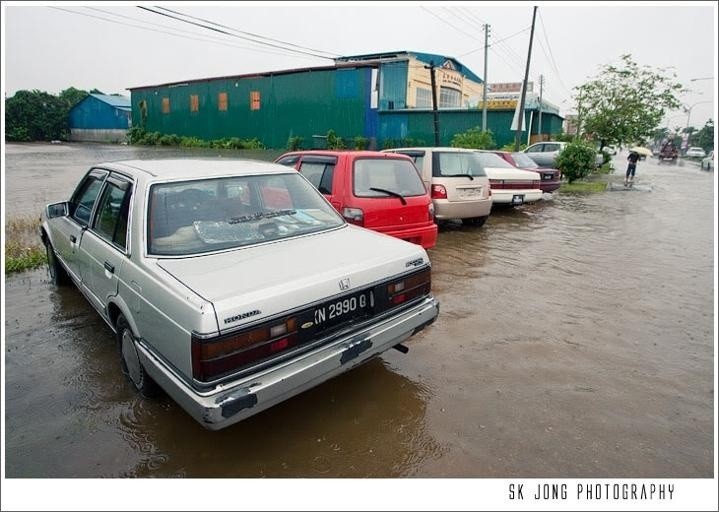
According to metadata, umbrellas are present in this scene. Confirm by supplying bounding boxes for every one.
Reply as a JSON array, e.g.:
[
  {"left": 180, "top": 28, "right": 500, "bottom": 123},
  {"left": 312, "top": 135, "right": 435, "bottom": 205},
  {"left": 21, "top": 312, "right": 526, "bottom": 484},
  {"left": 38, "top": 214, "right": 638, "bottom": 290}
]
[{"left": 630, "top": 146, "right": 653, "bottom": 156}]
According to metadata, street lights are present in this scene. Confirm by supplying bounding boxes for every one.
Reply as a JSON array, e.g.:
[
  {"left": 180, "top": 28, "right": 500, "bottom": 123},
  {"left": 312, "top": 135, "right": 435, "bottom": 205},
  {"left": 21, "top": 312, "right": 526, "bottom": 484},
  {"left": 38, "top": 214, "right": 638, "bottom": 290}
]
[{"left": 690, "top": 77, "right": 714, "bottom": 81}]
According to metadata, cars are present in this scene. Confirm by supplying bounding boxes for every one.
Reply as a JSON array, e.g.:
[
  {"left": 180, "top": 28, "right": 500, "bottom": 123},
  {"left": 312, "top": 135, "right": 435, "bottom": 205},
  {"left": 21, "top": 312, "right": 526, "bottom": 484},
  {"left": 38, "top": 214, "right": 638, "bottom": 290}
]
[
  {"left": 39, "top": 142, "right": 617, "bottom": 432},
  {"left": 653, "top": 138, "right": 714, "bottom": 171}
]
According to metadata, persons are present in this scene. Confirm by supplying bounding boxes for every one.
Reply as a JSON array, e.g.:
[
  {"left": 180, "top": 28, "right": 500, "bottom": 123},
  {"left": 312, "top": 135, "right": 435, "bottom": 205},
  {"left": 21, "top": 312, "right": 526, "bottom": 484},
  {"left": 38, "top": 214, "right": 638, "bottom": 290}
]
[{"left": 624, "top": 151, "right": 642, "bottom": 180}]
